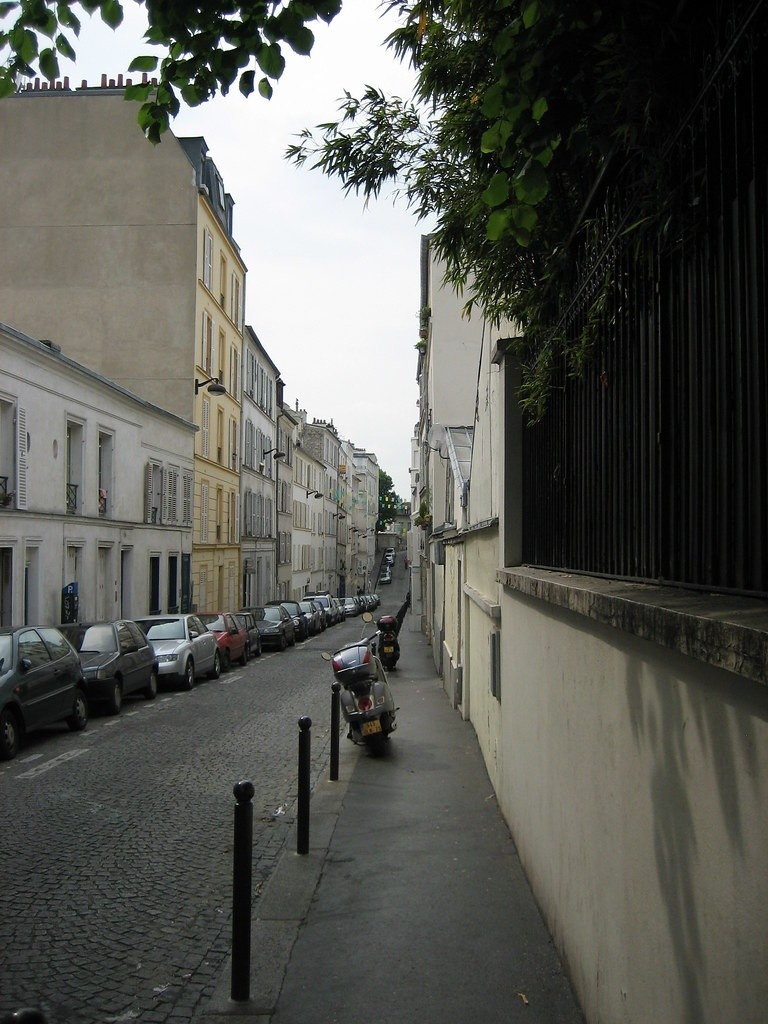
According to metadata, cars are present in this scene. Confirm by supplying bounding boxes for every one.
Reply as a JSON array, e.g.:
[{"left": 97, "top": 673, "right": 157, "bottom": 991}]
[
  {"left": 378, "top": 547, "right": 396, "bottom": 585},
  {"left": 265, "top": 599, "right": 308, "bottom": 640},
  {"left": 0, "top": 623, "right": 88, "bottom": 763},
  {"left": 193, "top": 611, "right": 250, "bottom": 673},
  {"left": 233, "top": 612, "right": 262, "bottom": 660},
  {"left": 239, "top": 604, "right": 296, "bottom": 652},
  {"left": 54, "top": 618, "right": 162, "bottom": 717},
  {"left": 297, "top": 590, "right": 381, "bottom": 638},
  {"left": 128, "top": 612, "right": 222, "bottom": 692}
]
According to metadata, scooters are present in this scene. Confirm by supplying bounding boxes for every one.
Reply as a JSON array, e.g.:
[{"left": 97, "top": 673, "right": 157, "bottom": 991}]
[
  {"left": 373, "top": 614, "right": 401, "bottom": 671},
  {"left": 321, "top": 611, "right": 401, "bottom": 759}
]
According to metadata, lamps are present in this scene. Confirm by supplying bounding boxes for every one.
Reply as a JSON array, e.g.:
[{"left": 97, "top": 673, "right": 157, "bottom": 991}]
[
  {"left": 358, "top": 533, "right": 368, "bottom": 538},
  {"left": 332, "top": 513, "right": 346, "bottom": 520},
  {"left": 263, "top": 447, "right": 286, "bottom": 462},
  {"left": 195, "top": 375, "right": 227, "bottom": 396},
  {"left": 349, "top": 526, "right": 359, "bottom": 533},
  {"left": 305, "top": 490, "right": 324, "bottom": 500}
]
